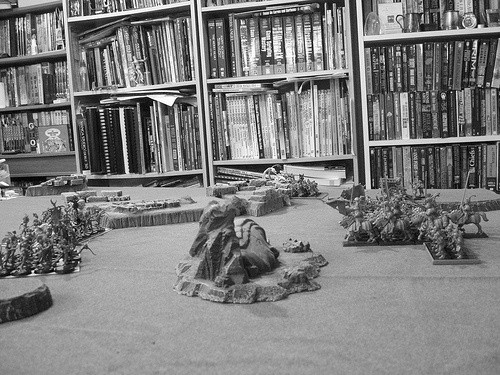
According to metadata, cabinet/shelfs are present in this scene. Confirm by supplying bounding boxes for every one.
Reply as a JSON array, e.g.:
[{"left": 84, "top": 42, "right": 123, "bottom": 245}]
[{"left": 0, "top": 0, "right": 500, "bottom": 190}]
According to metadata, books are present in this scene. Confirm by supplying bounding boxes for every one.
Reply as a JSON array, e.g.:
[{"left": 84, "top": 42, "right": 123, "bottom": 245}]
[{"left": 0, "top": 0, "right": 500, "bottom": 189}]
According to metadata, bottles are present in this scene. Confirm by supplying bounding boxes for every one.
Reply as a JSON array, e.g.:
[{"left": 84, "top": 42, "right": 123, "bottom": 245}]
[{"left": 443, "top": 10, "right": 461, "bottom": 30}]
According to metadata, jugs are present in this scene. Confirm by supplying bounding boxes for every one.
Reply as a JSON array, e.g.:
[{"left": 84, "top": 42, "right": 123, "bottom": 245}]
[{"left": 395, "top": 12, "right": 425, "bottom": 33}]
